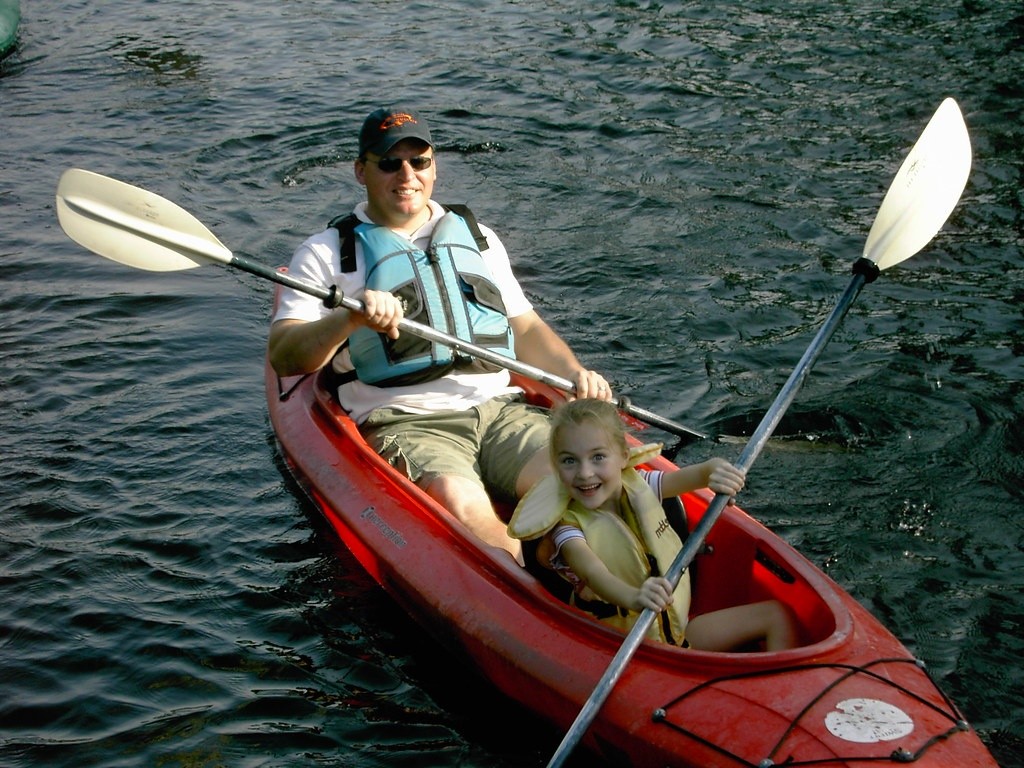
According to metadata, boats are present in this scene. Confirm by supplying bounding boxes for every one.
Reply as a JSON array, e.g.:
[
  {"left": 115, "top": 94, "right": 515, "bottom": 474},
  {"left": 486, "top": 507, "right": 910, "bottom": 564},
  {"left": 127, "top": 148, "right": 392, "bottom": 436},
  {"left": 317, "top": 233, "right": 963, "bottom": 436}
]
[{"left": 260, "top": 263, "right": 1000, "bottom": 767}]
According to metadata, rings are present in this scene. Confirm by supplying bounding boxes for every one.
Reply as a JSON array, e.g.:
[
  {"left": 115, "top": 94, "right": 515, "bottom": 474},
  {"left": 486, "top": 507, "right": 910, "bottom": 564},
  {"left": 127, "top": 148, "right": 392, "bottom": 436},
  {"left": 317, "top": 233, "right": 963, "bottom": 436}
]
[{"left": 600, "top": 387, "right": 605, "bottom": 390}]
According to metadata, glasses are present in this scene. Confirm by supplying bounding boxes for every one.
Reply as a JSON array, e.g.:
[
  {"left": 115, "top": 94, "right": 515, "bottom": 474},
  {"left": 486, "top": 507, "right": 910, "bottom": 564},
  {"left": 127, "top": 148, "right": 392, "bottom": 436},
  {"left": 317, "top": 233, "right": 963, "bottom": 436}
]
[{"left": 366, "top": 156, "right": 433, "bottom": 172}]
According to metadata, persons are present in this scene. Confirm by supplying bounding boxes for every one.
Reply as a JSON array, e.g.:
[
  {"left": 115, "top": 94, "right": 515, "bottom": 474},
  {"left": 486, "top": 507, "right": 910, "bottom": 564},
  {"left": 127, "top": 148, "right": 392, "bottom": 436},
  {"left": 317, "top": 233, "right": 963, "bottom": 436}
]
[
  {"left": 507, "top": 398, "right": 801, "bottom": 654},
  {"left": 269, "top": 109, "right": 612, "bottom": 563}
]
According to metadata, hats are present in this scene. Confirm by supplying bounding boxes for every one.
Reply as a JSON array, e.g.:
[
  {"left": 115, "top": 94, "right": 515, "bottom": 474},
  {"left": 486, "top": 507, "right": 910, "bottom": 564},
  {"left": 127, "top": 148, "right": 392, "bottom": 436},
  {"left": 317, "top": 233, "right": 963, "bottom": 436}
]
[{"left": 358, "top": 106, "right": 435, "bottom": 158}]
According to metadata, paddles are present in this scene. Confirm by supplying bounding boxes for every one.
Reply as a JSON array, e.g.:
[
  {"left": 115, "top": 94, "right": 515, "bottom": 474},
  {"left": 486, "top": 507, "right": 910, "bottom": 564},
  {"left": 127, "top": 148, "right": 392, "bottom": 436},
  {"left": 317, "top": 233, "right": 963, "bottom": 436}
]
[
  {"left": 56, "top": 167, "right": 843, "bottom": 455},
  {"left": 546, "top": 97, "right": 975, "bottom": 768}
]
